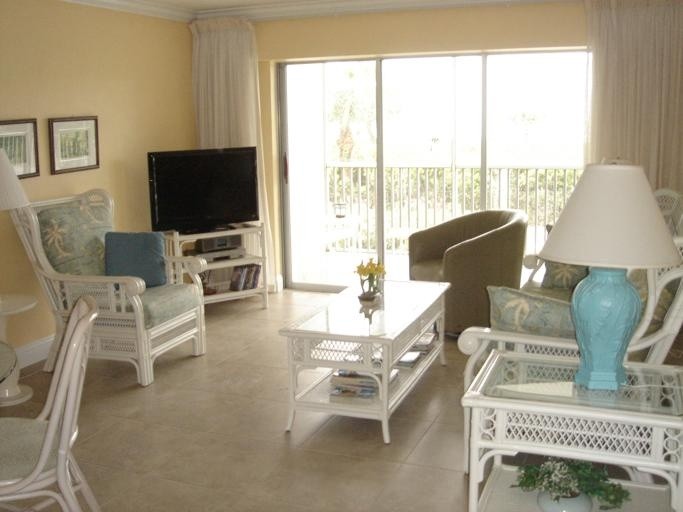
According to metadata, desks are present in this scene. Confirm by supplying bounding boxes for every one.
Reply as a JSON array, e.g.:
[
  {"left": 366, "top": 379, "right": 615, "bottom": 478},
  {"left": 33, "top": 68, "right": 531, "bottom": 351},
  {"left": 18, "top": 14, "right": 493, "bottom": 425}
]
[
  {"left": 0, "top": 293, "right": 41, "bottom": 408},
  {"left": 458, "top": 346, "right": 683, "bottom": 510}
]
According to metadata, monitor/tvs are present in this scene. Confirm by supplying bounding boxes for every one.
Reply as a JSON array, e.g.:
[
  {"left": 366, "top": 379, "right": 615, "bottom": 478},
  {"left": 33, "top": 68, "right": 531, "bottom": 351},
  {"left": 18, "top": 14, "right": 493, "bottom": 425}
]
[{"left": 148, "top": 146, "right": 260, "bottom": 235}]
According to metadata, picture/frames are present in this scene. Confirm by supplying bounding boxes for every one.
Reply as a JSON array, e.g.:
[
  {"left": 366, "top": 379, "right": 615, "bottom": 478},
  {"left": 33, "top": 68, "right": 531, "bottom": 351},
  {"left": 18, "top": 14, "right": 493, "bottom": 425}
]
[
  {"left": 0, "top": 116, "right": 41, "bottom": 181},
  {"left": 46, "top": 114, "right": 102, "bottom": 176}
]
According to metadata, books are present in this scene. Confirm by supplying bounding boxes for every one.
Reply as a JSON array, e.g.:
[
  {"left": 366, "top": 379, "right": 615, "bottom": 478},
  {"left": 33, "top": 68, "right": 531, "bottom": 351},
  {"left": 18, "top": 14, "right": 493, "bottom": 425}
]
[
  {"left": 328, "top": 332, "right": 437, "bottom": 405},
  {"left": 229, "top": 264, "right": 261, "bottom": 291}
]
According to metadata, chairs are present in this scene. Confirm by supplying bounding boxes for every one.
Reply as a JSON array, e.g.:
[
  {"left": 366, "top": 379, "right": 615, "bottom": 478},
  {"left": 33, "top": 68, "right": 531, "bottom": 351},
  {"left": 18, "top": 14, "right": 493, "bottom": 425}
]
[
  {"left": 8, "top": 187, "right": 208, "bottom": 388},
  {"left": 1, "top": 292, "right": 103, "bottom": 511}
]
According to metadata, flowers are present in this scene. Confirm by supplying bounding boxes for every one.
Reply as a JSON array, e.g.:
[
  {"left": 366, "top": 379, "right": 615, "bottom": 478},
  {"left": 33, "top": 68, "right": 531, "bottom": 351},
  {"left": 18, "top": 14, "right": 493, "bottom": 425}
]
[{"left": 354, "top": 258, "right": 385, "bottom": 288}]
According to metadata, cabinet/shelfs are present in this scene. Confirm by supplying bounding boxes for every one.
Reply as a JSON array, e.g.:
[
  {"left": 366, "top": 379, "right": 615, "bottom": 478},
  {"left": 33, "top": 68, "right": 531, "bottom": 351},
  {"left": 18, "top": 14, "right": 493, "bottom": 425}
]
[{"left": 163, "top": 219, "right": 269, "bottom": 317}]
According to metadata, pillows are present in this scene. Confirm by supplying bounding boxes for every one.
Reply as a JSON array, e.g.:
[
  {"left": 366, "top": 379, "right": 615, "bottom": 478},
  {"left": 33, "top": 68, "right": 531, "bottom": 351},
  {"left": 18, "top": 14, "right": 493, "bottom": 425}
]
[
  {"left": 33, "top": 188, "right": 116, "bottom": 295},
  {"left": 538, "top": 221, "right": 591, "bottom": 290},
  {"left": 103, "top": 226, "right": 168, "bottom": 288},
  {"left": 481, "top": 280, "right": 584, "bottom": 342}
]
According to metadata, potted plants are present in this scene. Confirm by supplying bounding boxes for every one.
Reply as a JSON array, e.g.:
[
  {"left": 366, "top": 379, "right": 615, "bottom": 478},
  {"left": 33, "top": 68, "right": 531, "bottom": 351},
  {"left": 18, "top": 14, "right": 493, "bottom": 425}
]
[{"left": 509, "top": 452, "right": 634, "bottom": 509}]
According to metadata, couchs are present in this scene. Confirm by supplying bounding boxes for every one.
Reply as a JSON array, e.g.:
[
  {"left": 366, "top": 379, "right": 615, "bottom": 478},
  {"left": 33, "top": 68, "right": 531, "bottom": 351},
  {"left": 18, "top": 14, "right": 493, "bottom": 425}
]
[
  {"left": 406, "top": 207, "right": 531, "bottom": 336},
  {"left": 455, "top": 187, "right": 682, "bottom": 375}
]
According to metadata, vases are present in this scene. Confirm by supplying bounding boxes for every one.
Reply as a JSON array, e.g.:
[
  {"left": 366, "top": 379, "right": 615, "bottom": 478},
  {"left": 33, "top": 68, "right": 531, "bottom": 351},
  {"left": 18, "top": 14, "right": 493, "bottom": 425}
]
[{"left": 358, "top": 289, "right": 381, "bottom": 306}]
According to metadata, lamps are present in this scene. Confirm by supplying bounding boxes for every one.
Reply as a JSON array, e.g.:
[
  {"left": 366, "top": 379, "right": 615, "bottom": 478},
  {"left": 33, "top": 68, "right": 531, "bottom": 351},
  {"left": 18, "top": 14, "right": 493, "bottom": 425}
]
[
  {"left": 0, "top": 147, "right": 36, "bottom": 214},
  {"left": 536, "top": 161, "right": 682, "bottom": 392}
]
